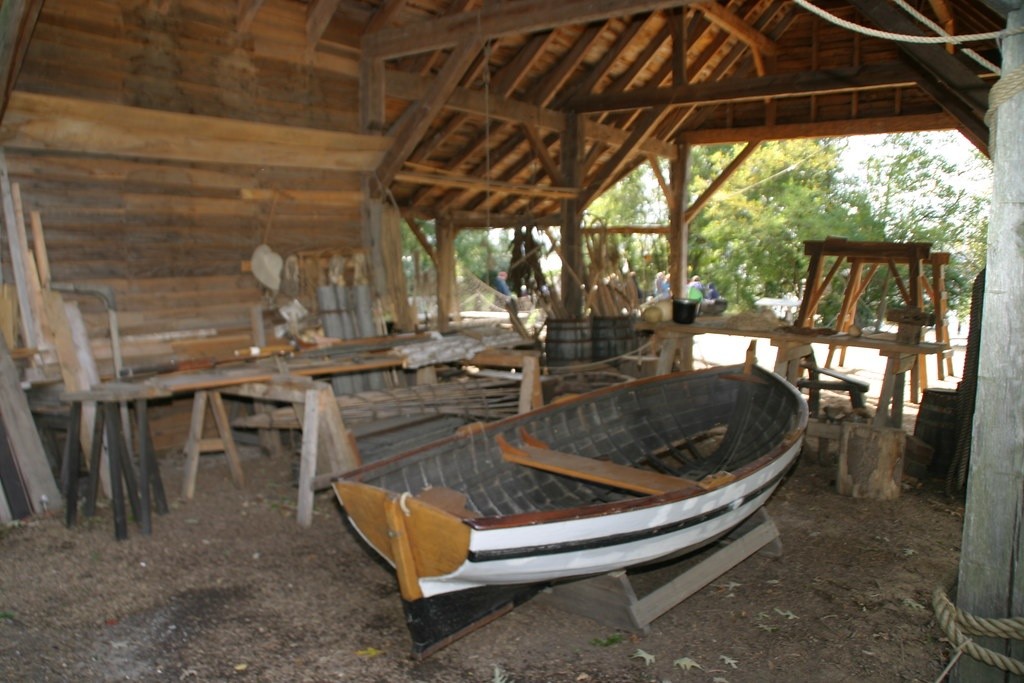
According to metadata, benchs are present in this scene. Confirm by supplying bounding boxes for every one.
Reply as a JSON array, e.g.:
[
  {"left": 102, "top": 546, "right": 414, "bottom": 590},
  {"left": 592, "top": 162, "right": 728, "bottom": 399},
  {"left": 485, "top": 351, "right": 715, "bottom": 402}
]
[{"left": 497, "top": 426, "right": 698, "bottom": 497}]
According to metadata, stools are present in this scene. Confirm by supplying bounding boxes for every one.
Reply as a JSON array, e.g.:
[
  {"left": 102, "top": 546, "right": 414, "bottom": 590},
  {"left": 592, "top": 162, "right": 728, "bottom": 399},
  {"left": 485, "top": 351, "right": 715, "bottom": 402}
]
[{"left": 58, "top": 381, "right": 174, "bottom": 543}]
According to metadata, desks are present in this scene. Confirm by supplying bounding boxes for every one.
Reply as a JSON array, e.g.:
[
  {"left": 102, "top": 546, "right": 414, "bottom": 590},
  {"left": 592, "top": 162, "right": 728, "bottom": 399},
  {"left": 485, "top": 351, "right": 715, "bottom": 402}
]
[{"left": 631, "top": 315, "right": 950, "bottom": 429}]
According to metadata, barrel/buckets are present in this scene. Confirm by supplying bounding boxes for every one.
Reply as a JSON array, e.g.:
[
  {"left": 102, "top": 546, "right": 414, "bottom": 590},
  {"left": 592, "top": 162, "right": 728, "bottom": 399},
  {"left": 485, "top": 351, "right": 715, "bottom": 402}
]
[
  {"left": 591, "top": 314, "right": 638, "bottom": 365},
  {"left": 915, "top": 388, "right": 957, "bottom": 465},
  {"left": 547, "top": 318, "right": 590, "bottom": 366}
]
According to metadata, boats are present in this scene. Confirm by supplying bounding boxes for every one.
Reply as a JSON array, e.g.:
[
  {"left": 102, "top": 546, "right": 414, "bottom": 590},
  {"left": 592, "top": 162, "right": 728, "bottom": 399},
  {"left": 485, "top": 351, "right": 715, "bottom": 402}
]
[{"left": 327, "top": 338, "right": 810, "bottom": 662}]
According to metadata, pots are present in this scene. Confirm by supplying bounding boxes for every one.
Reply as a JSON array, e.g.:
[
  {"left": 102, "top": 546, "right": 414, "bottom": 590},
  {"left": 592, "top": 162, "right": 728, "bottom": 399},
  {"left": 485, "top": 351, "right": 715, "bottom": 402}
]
[{"left": 669, "top": 295, "right": 702, "bottom": 323}]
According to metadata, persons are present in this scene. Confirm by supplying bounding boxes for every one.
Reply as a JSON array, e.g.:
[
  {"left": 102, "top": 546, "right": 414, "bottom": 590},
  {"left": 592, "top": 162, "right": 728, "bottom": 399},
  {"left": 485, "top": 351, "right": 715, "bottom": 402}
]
[
  {"left": 705, "top": 282, "right": 719, "bottom": 299},
  {"left": 495, "top": 272, "right": 510, "bottom": 295},
  {"left": 688, "top": 276, "right": 704, "bottom": 292},
  {"left": 655, "top": 272, "right": 670, "bottom": 295},
  {"left": 623, "top": 272, "right": 644, "bottom": 297}
]
